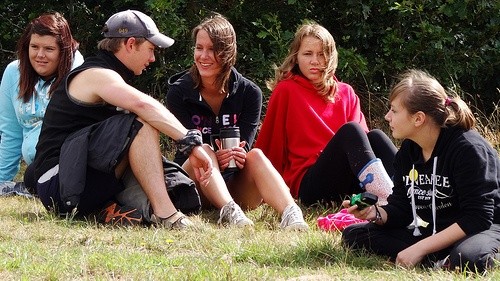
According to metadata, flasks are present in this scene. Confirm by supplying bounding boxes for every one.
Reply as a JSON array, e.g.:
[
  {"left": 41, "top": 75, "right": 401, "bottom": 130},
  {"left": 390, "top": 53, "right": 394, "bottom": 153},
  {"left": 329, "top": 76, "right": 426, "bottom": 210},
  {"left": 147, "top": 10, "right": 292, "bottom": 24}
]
[{"left": 210, "top": 127, "right": 240, "bottom": 168}]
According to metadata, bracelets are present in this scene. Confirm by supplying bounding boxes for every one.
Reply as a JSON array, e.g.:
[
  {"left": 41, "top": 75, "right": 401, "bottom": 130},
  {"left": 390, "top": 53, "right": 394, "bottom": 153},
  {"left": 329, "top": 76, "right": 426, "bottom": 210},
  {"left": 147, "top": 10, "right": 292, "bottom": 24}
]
[{"left": 366, "top": 209, "right": 380, "bottom": 224}]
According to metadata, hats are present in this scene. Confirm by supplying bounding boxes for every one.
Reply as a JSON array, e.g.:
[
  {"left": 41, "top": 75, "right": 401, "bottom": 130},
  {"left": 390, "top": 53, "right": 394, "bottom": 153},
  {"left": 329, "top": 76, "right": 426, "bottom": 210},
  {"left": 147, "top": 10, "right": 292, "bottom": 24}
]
[{"left": 100, "top": 9, "right": 175, "bottom": 49}]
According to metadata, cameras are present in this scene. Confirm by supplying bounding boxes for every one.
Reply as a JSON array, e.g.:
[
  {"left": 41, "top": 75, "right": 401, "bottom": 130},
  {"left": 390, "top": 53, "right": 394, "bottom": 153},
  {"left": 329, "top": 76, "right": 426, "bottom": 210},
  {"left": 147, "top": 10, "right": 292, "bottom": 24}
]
[{"left": 352, "top": 193, "right": 378, "bottom": 210}]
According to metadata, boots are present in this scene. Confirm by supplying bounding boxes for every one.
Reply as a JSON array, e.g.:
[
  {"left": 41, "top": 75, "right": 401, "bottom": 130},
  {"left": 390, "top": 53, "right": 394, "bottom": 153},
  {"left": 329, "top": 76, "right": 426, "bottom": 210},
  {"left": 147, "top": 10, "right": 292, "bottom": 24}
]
[{"left": 358, "top": 157, "right": 394, "bottom": 206}]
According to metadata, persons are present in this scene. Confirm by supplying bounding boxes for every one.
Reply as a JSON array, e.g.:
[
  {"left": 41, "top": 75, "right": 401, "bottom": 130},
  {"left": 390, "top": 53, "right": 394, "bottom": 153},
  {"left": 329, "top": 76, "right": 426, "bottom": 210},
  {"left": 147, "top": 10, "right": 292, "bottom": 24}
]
[
  {"left": 166, "top": 13, "right": 309, "bottom": 231},
  {"left": 34, "top": 11, "right": 213, "bottom": 230},
  {"left": 252, "top": 19, "right": 399, "bottom": 207},
  {"left": 341, "top": 72, "right": 500, "bottom": 273},
  {"left": 0, "top": 12, "right": 84, "bottom": 198}
]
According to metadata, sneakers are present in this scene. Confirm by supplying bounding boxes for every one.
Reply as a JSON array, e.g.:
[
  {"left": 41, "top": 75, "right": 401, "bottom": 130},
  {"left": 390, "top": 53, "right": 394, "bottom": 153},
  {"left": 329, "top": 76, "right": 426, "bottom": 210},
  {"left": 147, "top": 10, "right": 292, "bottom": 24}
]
[
  {"left": 216, "top": 200, "right": 255, "bottom": 228},
  {"left": 280, "top": 203, "right": 311, "bottom": 232}
]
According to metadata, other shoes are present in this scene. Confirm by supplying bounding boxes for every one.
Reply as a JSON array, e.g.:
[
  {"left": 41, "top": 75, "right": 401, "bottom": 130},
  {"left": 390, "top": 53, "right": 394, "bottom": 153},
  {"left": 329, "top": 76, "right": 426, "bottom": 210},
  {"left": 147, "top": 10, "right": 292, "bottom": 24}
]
[
  {"left": 150, "top": 209, "right": 196, "bottom": 231},
  {"left": 97, "top": 201, "right": 143, "bottom": 230}
]
